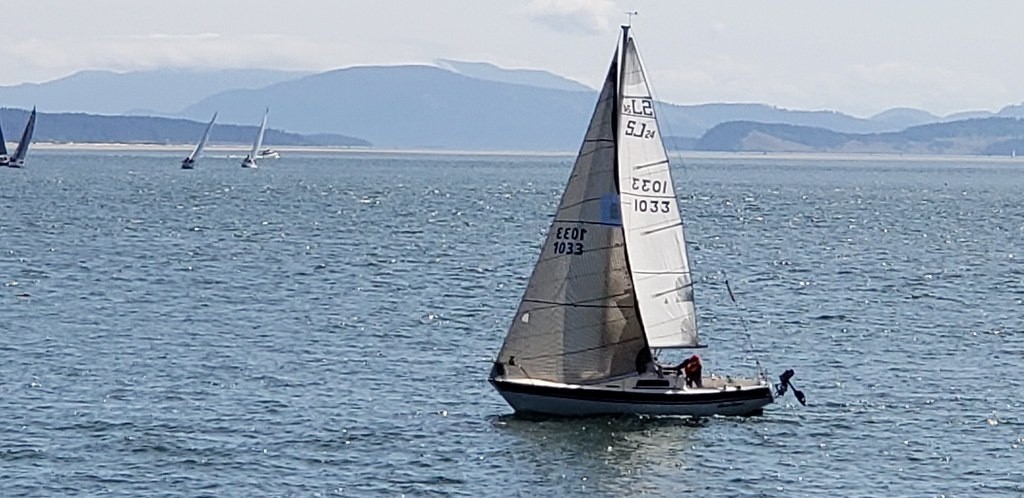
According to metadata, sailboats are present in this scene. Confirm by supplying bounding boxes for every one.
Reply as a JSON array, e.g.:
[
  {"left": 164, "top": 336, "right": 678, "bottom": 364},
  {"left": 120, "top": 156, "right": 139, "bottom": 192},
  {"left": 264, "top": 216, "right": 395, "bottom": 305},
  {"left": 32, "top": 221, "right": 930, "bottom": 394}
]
[
  {"left": 487, "top": 23, "right": 807, "bottom": 418},
  {"left": 241, "top": 106, "right": 269, "bottom": 169},
  {"left": 181, "top": 111, "right": 218, "bottom": 170},
  {"left": 9, "top": 105, "right": 37, "bottom": 168},
  {"left": 0, "top": 125, "right": 11, "bottom": 167}
]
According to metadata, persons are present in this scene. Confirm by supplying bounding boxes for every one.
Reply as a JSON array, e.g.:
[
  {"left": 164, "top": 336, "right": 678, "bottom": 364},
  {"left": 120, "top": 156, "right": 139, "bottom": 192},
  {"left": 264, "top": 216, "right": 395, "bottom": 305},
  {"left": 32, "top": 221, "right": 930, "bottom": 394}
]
[{"left": 676, "top": 355, "right": 703, "bottom": 388}]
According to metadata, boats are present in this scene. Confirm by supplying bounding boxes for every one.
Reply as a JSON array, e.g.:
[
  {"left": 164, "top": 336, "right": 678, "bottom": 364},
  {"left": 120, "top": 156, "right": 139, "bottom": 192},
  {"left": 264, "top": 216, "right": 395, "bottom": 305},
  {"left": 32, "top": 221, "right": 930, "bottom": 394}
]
[{"left": 255, "top": 149, "right": 279, "bottom": 158}]
